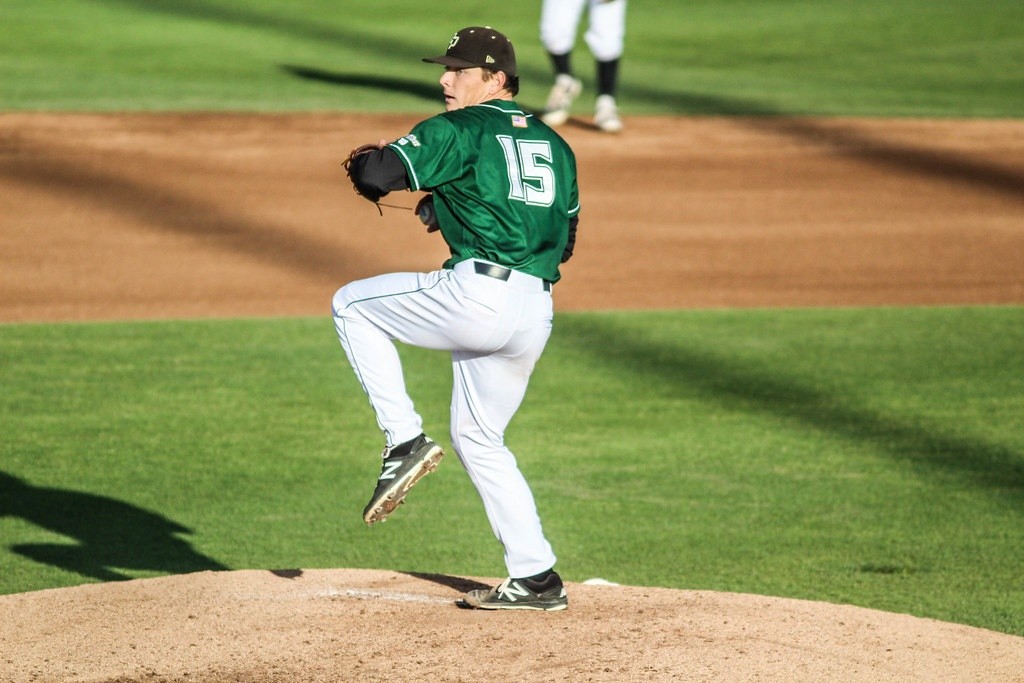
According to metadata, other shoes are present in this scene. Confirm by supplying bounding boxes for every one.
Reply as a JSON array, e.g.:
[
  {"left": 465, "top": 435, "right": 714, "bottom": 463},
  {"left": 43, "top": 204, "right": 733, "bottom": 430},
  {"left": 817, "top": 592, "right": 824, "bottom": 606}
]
[
  {"left": 542, "top": 74, "right": 584, "bottom": 126},
  {"left": 593, "top": 94, "right": 623, "bottom": 132}
]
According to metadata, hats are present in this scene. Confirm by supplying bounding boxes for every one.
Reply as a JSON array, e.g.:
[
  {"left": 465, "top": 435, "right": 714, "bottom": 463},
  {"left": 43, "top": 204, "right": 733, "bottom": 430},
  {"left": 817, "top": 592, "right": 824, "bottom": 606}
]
[{"left": 422, "top": 26, "right": 516, "bottom": 76}]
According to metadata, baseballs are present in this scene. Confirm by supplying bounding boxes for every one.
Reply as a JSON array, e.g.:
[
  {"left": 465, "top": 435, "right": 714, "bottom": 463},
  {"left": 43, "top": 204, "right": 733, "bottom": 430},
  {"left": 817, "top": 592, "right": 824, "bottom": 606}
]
[{"left": 418, "top": 202, "right": 437, "bottom": 226}]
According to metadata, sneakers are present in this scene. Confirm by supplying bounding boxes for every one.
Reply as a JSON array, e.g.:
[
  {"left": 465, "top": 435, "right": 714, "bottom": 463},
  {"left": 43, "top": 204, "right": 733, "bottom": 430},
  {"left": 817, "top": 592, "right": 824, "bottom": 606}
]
[
  {"left": 362, "top": 433, "right": 445, "bottom": 523},
  {"left": 463, "top": 572, "right": 568, "bottom": 611}
]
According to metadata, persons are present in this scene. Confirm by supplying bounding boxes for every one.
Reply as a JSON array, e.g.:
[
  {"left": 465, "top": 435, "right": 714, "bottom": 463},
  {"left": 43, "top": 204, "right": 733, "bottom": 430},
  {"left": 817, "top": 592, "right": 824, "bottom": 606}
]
[
  {"left": 331, "top": 27, "right": 580, "bottom": 611},
  {"left": 539, "top": 0, "right": 627, "bottom": 132}
]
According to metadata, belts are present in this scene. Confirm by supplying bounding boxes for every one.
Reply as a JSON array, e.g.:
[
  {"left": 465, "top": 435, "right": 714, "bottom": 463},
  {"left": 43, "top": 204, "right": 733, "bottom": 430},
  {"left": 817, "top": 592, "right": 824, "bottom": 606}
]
[{"left": 474, "top": 261, "right": 551, "bottom": 291}]
